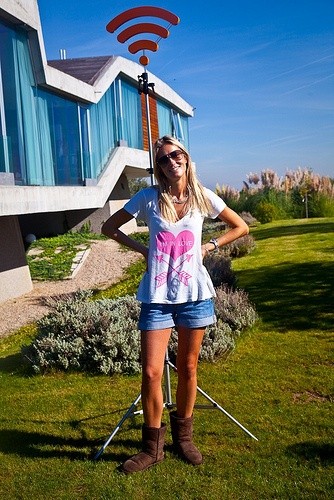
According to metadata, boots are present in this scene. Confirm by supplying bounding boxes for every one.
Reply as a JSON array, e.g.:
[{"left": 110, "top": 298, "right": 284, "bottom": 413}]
[
  {"left": 123, "top": 422, "right": 167, "bottom": 474},
  {"left": 169, "top": 411, "right": 204, "bottom": 466}
]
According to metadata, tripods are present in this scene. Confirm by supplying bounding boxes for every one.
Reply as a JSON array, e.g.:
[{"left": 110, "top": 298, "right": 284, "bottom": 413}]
[{"left": 95, "top": 91, "right": 263, "bottom": 457}]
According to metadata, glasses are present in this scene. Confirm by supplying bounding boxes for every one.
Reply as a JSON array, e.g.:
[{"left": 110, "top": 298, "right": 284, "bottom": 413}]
[{"left": 156, "top": 149, "right": 185, "bottom": 167}]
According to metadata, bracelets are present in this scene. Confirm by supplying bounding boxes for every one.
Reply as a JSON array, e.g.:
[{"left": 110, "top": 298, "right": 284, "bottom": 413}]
[
  {"left": 201, "top": 246, "right": 209, "bottom": 253},
  {"left": 209, "top": 238, "right": 219, "bottom": 249}
]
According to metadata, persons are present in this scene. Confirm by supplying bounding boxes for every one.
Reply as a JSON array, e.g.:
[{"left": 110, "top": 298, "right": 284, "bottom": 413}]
[{"left": 101, "top": 137, "right": 251, "bottom": 473}]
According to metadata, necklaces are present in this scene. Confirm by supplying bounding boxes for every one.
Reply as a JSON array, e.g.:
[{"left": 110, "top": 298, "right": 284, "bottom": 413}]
[{"left": 168, "top": 186, "right": 191, "bottom": 203}]
